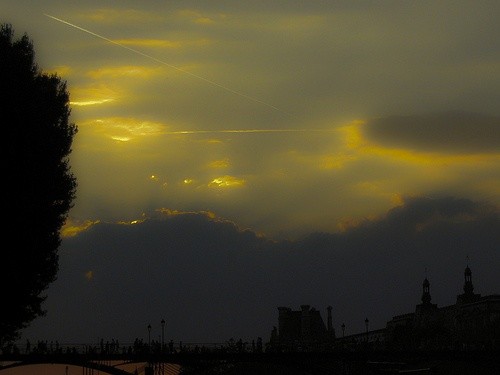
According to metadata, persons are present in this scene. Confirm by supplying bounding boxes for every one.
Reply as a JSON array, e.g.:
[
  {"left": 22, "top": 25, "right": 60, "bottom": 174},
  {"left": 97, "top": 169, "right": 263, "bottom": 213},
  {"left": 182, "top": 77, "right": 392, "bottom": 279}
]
[{"left": 0, "top": 337, "right": 500, "bottom": 357}]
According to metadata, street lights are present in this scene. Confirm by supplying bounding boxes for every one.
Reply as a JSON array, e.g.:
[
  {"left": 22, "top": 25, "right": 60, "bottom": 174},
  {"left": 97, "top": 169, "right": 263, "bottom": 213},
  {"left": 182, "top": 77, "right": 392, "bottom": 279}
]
[
  {"left": 341, "top": 323, "right": 345, "bottom": 353},
  {"left": 160, "top": 318, "right": 165, "bottom": 351},
  {"left": 148, "top": 323, "right": 151, "bottom": 354},
  {"left": 365, "top": 318, "right": 369, "bottom": 345}
]
